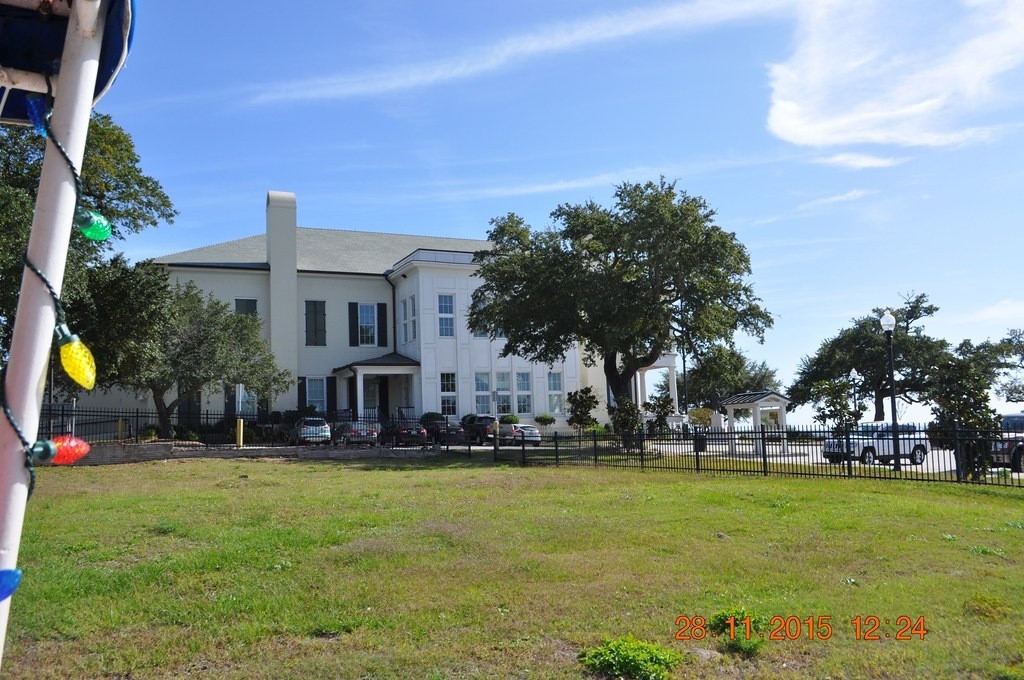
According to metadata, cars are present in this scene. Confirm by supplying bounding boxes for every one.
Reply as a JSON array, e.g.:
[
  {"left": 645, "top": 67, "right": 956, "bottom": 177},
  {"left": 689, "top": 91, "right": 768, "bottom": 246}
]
[
  {"left": 288, "top": 417, "right": 331, "bottom": 444},
  {"left": 427, "top": 421, "right": 464, "bottom": 444},
  {"left": 378, "top": 421, "right": 427, "bottom": 444},
  {"left": 332, "top": 422, "right": 377, "bottom": 447},
  {"left": 499, "top": 424, "right": 541, "bottom": 446}
]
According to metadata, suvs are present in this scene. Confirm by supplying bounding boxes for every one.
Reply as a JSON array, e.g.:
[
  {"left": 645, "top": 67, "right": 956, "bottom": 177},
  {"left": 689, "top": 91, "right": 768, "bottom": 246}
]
[
  {"left": 961, "top": 414, "right": 1024, "bottom": 472},
  {"left": 822, "top": 421, "right": 931, "bottom": 466},
  {"left": 460, "top": 413, "right": 500, "bottom": 445}
]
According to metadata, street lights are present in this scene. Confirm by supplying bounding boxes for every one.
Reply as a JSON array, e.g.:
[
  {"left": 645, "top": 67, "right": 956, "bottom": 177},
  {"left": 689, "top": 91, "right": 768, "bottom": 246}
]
[{"left": 880, "top": 309, "right": 901, "bottom": 471}]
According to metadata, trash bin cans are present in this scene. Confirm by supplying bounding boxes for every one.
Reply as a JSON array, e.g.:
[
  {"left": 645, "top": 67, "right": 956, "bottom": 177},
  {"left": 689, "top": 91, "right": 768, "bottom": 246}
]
[{"left": 693, "top": 435, "right": 707, "bottom": 452}]
